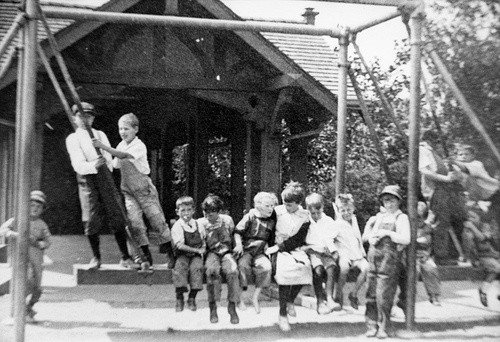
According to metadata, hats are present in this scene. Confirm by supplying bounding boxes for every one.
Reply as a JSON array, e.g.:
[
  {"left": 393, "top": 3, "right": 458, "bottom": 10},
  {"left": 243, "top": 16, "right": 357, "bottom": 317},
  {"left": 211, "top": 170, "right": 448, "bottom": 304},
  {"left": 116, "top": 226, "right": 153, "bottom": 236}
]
[
  {"left": 71, "top": 102, "right": 97, "bottom": 115},
  {"left": 379, "top": 185, "right": 403, "bottom": 202},
  {"left": 30, "top": 190, "right": 47, "bottom": 207}
]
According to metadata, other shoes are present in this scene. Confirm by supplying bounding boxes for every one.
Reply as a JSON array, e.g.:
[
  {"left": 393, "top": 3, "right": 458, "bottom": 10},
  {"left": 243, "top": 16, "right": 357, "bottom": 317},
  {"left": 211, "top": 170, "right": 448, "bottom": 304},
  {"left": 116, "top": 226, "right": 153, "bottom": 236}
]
[
  {"left": 89, "top": 258, "right": 102, "bottom": 270},
  {"left": 208, "top": 305, "right": 218, "bottom": 323},
  {"left": 119, "top": 257, "right": 136, "bottom": 268},
  {"left": 365, "top": 322, "right": 387, "bottom": 339},
  {"left": 429, "top": 294, "right": 440, "bottom": 306},
  {"left": 137, "top": 255, "right": 153, "bottom": 266},
  {"left": 167, "top": 252, "right": 177, "bottom": 268},
  {"left": 479, "top": 287, "right": 489, "bottom": 307},
  {"left": 278, "top": 315, "right": 292, "bottom": 332},
  {"left": 349, "top": 292, "right": 359, "bottom": 309},
  {"left": 228, "top": 302, "right": 239, "bottom": 324},
  {"left": 187, "top": 298, "right": 197, "bottom": 311},
  {"left": 175, "top": 299, "right": 186, "bottom": 311},
  {"left": 288, "top": 303, "right": 297, "bottom": 318},
  {"left": 317, "top": 299, "right": 341, "bottom": 316}
]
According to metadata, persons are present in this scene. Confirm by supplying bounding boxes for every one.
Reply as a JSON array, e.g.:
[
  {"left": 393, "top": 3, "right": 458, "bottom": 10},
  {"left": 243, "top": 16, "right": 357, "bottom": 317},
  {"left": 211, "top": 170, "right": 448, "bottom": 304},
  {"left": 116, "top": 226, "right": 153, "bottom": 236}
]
[
  {"left": 0, "top": 189, "right": 53, "bottom": 324},
  {"left": 91, "top": 113, "right": 176, "bottom": 269},
  {"left": 171, "top": 132, "right": 500, "bottom": 338},
  {"left": 65, "top": 102, "right": 141, "bottom": 271}
]
